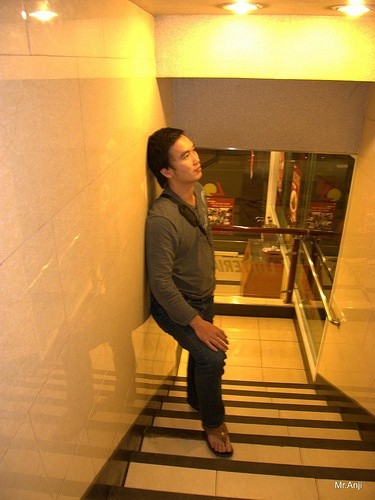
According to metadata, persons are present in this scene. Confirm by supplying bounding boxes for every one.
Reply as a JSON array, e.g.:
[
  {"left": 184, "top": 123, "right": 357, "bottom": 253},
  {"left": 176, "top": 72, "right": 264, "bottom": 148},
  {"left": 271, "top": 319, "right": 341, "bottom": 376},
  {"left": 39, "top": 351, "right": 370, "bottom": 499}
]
[{"left": 146, "top": 127, "right": 235, "bottom": 458}]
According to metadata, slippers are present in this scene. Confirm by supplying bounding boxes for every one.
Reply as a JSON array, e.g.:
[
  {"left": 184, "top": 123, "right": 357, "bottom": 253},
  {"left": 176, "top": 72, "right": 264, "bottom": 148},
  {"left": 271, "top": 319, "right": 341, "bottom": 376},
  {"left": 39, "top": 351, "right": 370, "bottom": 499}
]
[{"left": 202, "top": 423, "right": 233, "bottom": 457}]
